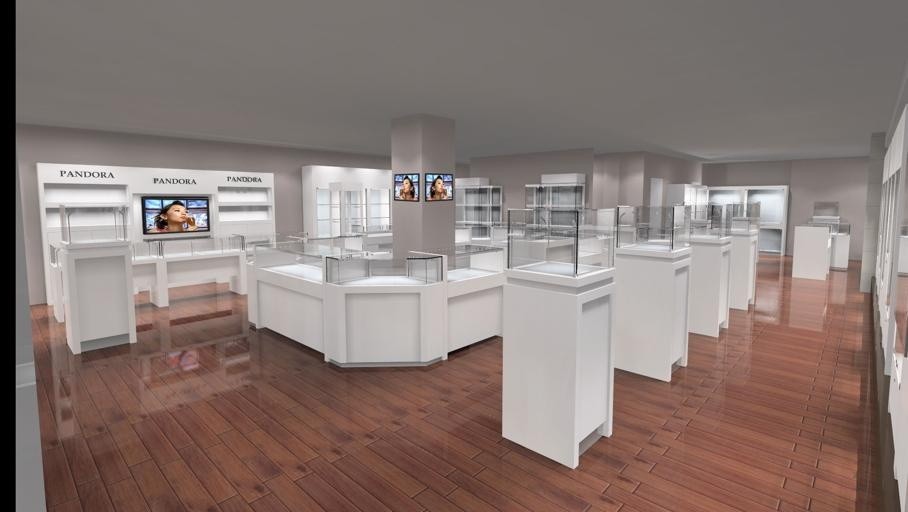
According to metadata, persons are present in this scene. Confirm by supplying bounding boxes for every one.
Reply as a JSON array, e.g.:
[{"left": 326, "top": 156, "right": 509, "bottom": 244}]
[
  {"left": 427, "top": 175, "right": 449, "bottom": 200},
  {"left": 394, "top": 175, "right": 417, "bottom": 200},
  {"left": 147, "top": 200, "right": 199, "bottom": 234}
]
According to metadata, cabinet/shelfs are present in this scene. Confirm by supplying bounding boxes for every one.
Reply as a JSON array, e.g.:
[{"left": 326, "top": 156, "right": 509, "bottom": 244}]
[{"left": 304, "top": 179, "right": 587, "bottom": 246}]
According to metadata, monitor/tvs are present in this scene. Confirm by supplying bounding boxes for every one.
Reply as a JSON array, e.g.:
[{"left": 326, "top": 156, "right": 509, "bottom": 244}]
[
  {"left": 424, "top": 172, "right": 454, "bottom": 202},
  {"left": 140, "top": 193, "right": 212, "bottom": 240},
  {"left": 393, "top": 172, "right": 420, "bottom": 203}
]
